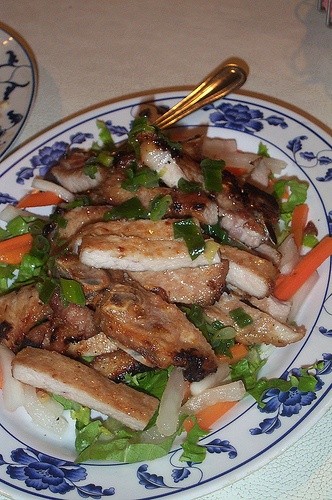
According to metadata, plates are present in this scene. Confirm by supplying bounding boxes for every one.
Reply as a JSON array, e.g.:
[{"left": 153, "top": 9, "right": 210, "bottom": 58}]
[
  {"left": 0, "top": 25, "right": 38, "bottom": 161},
  {"left": 0, "top": 87, "right": 331, "bottom": 500}
]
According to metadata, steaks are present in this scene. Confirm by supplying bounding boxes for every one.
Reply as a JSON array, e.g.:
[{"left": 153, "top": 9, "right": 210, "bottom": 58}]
[{"left": 0, "top": 100, "right": 332, "bottom": 466}]
